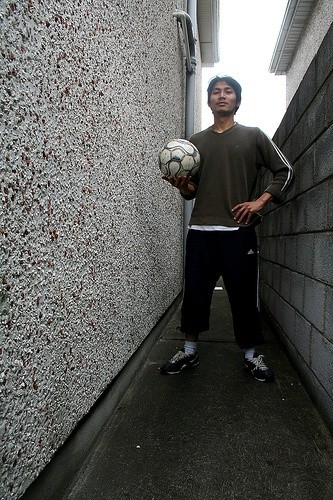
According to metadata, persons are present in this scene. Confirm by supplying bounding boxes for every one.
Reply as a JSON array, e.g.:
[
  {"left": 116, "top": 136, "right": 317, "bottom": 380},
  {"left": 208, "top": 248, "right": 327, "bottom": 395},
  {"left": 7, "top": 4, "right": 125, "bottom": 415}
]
[{"left": 158, "top": 77, "right": 295, "bottom": 382}]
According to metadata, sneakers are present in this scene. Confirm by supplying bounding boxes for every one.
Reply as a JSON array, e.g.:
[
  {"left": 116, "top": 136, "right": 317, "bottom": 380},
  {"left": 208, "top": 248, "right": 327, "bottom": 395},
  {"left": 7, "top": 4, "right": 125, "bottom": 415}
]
[
  {"left": 159, "top": 348, "right": 199, "bottom": 375},
  {"left": 241, "top": 348, "right": 274, "bottom": 382}
]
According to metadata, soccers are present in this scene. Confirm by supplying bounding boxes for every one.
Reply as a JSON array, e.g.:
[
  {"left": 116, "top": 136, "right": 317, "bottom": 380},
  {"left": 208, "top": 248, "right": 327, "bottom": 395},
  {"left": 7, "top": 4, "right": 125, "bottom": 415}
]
[{"left": 160, "top": 139, "right": 199, "bottom": 179}]
